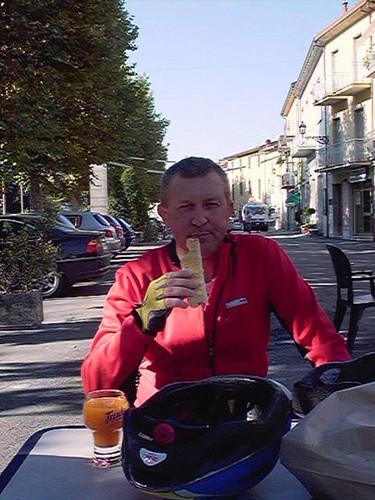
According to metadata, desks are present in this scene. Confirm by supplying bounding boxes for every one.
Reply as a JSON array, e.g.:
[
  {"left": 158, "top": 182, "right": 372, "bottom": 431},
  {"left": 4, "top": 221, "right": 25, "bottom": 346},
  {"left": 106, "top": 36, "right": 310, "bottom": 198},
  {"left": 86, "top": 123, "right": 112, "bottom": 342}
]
[{"left": 0, "top": 419, "right": 314, "bottom": 500}]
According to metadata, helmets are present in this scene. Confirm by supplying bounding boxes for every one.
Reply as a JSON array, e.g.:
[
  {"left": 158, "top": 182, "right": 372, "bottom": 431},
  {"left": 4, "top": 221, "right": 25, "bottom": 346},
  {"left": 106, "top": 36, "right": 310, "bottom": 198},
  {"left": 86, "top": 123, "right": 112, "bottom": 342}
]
[{"left": 122, "top": 373, "right": 305, "bottom": 500}]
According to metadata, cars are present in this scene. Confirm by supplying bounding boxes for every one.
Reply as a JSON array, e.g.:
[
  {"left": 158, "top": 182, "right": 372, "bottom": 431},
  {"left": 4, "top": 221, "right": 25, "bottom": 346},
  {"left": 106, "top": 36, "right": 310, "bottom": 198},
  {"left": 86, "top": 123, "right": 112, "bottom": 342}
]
[{"left": 0, "top": 201, "right": 139, "bottom": 299}]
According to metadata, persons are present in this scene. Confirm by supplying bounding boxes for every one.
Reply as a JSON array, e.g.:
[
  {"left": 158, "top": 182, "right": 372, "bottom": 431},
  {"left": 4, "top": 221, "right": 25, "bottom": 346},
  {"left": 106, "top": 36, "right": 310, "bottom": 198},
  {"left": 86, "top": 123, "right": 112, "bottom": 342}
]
[{"left": 81, "top": 156, "right": 352, "bottom": 407}]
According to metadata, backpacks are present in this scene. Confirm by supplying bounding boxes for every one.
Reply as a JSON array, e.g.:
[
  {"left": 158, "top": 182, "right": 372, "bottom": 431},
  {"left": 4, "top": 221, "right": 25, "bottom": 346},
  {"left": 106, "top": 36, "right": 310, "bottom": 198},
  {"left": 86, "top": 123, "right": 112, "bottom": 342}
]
[{"left": 290, "top": 351, "right": 375, "bottom": 419}]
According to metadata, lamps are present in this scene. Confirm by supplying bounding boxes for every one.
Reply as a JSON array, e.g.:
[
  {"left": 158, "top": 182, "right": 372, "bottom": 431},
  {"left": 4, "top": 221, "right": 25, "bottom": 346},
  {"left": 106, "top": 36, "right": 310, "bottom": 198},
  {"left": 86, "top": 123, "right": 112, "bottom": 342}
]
[{"left": 299, "top": 121, "right": 329, "bottom": 145}]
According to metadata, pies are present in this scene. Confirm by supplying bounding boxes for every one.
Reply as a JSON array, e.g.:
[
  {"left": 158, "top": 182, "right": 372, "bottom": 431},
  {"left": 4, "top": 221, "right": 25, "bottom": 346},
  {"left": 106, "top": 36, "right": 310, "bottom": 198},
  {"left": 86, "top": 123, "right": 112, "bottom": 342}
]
[{"left": 180, "top": 237, "right": 209, "bottom": 308}]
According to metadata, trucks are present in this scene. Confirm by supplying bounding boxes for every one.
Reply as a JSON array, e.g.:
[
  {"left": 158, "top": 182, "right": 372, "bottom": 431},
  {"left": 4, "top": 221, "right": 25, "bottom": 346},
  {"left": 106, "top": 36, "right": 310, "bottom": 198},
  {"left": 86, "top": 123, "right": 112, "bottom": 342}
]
[{"left": 228, "top": 201, "right": 277, "bottom": 234}]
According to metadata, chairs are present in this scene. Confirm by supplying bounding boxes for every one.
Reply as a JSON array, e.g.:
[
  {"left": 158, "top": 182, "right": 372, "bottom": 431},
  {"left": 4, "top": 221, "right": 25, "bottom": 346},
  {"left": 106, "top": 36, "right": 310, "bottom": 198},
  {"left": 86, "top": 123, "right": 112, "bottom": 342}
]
[{"left": 325, "top": 244, "right": 375, "bottom": 354}]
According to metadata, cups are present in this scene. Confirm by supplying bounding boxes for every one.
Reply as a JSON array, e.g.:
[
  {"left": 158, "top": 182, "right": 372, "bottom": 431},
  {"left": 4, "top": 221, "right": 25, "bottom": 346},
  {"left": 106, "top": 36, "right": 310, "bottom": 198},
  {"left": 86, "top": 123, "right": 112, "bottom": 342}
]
[{"left": 82, "top": 388, "right": 130, "bottom": 468}]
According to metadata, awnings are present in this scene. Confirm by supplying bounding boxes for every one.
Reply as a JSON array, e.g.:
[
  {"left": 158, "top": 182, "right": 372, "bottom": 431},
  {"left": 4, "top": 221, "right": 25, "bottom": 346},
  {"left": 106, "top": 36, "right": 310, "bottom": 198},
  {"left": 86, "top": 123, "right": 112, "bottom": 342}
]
[{"left": 285, "top": 193, "right": 300, "bottom": 205}]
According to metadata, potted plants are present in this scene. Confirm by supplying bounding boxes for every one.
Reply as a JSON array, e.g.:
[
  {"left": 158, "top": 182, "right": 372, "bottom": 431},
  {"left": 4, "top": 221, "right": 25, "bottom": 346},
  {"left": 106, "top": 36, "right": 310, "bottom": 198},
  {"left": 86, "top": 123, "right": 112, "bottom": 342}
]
[{"left": 0, "top": 190, "right": 65, "bottom": 331}]
